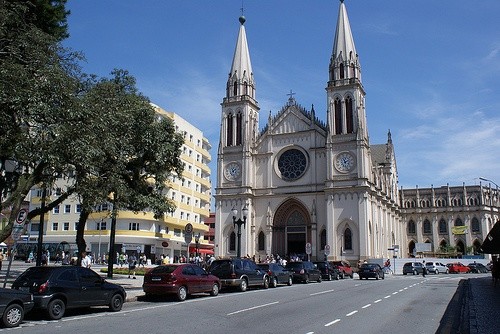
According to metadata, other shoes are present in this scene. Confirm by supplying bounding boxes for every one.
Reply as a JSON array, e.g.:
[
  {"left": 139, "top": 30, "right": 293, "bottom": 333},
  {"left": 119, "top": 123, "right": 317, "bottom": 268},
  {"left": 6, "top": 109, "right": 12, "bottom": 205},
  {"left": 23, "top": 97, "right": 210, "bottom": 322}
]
[
  {"left": 128, "top": 275, "right": 130, "bottom": 278},
  {"left": 133, "top": 277, "right": 136, "bottom": 279}
]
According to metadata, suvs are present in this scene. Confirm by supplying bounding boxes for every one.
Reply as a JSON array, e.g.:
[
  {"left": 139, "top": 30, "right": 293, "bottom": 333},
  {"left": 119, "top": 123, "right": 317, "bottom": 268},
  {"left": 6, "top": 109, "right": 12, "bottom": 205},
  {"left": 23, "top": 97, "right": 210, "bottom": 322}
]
[
  {"left": 448, "top": 262, "right": 471, "bottom": 274},
  {"left": 208, "top": 258, "right": 271, "bottom": 292},
  {"left": 425, "top": 261, "right": 449, "bottom": 275}
]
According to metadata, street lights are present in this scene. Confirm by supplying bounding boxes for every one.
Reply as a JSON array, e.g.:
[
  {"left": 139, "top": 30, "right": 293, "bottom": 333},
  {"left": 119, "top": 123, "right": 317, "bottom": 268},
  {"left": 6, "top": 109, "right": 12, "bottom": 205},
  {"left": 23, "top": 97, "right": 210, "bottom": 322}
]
[{"left": 231, "top": 203, "right": 248, "bottom": 260}]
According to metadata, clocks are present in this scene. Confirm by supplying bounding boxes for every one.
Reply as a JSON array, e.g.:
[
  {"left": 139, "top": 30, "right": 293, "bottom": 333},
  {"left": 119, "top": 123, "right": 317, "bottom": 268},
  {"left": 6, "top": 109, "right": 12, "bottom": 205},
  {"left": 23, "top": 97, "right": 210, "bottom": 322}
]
[
  {"left": 333, "top": 150, "right": 357, "bottom": 174},
  {"left": 223, "top": 161, "right": 242, "bottom": 182}
]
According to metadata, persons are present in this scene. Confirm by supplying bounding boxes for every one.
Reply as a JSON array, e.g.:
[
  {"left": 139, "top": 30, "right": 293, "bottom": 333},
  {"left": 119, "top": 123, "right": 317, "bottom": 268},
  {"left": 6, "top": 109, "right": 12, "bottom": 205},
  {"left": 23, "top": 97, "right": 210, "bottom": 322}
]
[
  {"left": 138, "top": 253, "right": 147, "bottom": 268},
  {"left": 243, "top": 253, "right": 303, "bottom": 267},
  {"left": 41, "top": 245, "right": 77, "bottom": 266},
  {"left": 385, "top": 259, "right": 390, "bottom": 267},
  {"left": 81, "top": 251, "right": 91, "bottom": 269},
  {"left": 363, "top": 259, "right": 367, "bottom": 263},
  {"left": 421, "top": 260, "right": 428, "bottom": 277},
  {"left": 357, "top": 260, "right": 362, "bottom": 273},
  {"left": 88, "top": 250, "right": 128, "bottom": 267},
  {"left": 0, "top": 243, "right": 37, "bottom": 272},
  {"left": 127, "top": 250, "right": 136, "bottom": 279},
  {"left": 473, "top": 260, "right": 476, "bottom": 264},
  {"left": 150, "top": 252, "right": 233, "bottom": 269},
  {"left": 459, "top": 263, "right": 463, "bottom": 274}
]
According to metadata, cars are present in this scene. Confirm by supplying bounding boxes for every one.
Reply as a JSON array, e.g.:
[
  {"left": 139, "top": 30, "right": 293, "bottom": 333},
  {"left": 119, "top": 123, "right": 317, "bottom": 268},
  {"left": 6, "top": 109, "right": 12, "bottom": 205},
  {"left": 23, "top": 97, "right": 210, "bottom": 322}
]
[
  {"left": 312, "top": 260, "right": 340, "bottom": 281},
  {"left": 142, "top": 262, "right": 223, "bottom": 301},
  {"left": 358, "top": 263, "right": 384, "bottom": 280},
  {"left": 402, "top": 261, "right": 429, "bottom": 275},
  {"left": 10, "top": 264, "right": 127, "bottom": 321},
  {"left": 467, "top": 262, "right": 488, "bottom": 274},
  {"left": 256, "top": 263, "right": 293, "bottom": 288},
  {"left": 484, "top": 263, "right": 493, "bottom": 271},
  {"left": 327, "top": 259, "right": 353, "bottom": 279},
  {"left": 0, "top": 287, "right": 35, "bottom": 328},
  {"left": 283, "top": 261, "right": 322, "bottom": 284}
]
